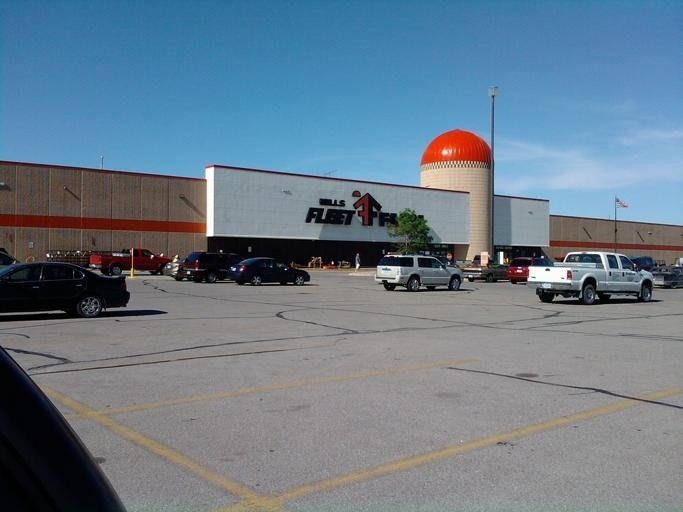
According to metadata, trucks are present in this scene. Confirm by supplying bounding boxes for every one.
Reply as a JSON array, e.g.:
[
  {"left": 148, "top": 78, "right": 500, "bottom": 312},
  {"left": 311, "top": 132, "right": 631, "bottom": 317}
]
[{"left": 463, "top": 254, "right": 508, "bottom": 282}]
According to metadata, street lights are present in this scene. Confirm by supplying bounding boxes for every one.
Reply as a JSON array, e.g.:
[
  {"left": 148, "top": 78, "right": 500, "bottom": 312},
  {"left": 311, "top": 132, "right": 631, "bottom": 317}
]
[{"left": 489, "top": 84, "right": 497, "bottom": 259}]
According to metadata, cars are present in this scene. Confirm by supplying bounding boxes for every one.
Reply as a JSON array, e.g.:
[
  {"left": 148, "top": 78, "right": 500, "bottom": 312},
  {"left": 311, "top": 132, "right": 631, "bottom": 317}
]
[
  {"left": 0, "top": 344, "right": 124, "bottom": 510},
  {"left": 164, "top": 251, "right": 310, "bottom": 286},
  {"left": 0, "top": 263, "right": 131, "bottom": 317}
]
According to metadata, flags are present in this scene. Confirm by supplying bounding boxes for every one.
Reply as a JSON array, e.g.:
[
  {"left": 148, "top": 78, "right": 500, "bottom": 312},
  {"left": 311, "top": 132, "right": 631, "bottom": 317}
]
[{"left": 615, "top": 197, "right": 628, "bottom": 209}]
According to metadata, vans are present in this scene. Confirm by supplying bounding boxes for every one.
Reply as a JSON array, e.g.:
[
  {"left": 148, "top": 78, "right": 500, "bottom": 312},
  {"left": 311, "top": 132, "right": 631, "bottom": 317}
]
[
  {"left": 508, "top": 257, "right": 550, "bottom": 285},
  {"left": 631, "top": 256, "right": 653, "bottom": 270}
]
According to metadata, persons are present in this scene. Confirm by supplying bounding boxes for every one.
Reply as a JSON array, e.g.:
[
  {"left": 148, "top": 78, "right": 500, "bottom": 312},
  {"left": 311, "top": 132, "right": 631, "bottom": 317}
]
[{"left": 354, "top": 253, "right": 361, "bottom": 273}]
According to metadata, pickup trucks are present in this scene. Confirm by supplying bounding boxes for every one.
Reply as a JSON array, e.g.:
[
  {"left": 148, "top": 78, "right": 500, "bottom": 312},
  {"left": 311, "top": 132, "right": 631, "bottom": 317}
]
[
  {"left": 90, "top": 247, "right": 172, "bottom": 276},
  {"left": 528, "top": 251, "right": 655, "bottom": 304}
]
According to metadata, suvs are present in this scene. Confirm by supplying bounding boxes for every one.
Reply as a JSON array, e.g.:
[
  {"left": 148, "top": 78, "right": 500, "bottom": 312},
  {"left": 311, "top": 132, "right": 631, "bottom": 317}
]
[{"left": 375, "top": 254, "right": 465, "bottom": 290}]
